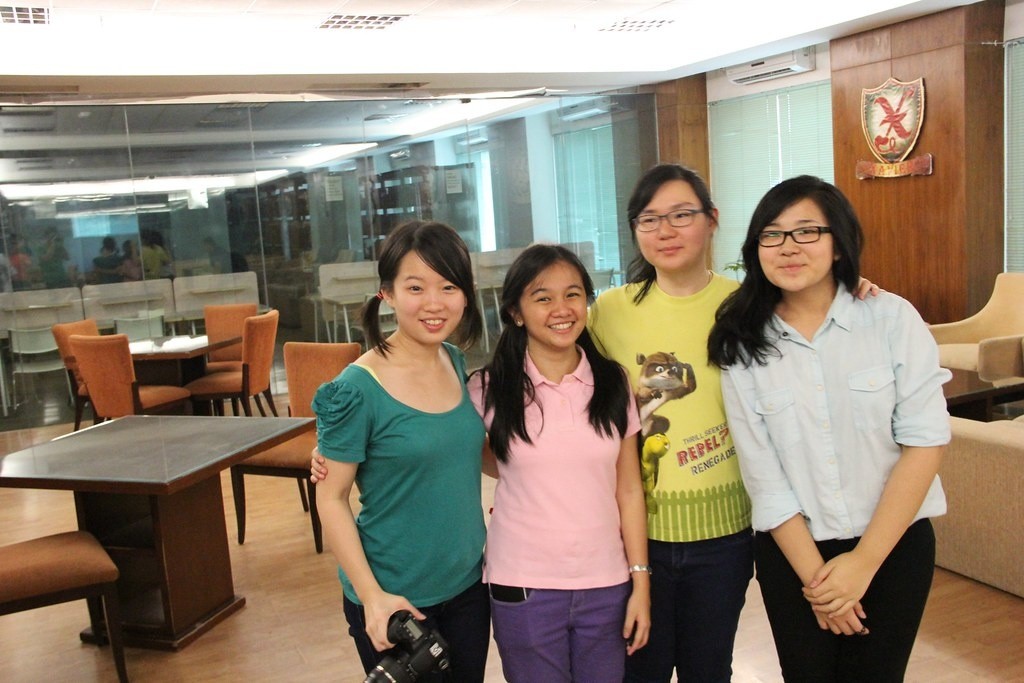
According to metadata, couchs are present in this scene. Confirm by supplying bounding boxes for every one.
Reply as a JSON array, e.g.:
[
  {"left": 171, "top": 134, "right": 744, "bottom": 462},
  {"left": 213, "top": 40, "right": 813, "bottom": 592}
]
[
  {"left": 927, "top": 272, "right": 1024, "bottom": 380},
  {"left": 927, "top": 412, "right": 1024, "bottom": 599}
]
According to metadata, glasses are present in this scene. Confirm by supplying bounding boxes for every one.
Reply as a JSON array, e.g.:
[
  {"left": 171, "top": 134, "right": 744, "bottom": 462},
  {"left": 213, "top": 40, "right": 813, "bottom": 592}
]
[
  {"left": 755, "top": 225, "right": 832, "bottom": 247},
  {"left": 632, "top": 208, "right": 705, "bottom": 232}
]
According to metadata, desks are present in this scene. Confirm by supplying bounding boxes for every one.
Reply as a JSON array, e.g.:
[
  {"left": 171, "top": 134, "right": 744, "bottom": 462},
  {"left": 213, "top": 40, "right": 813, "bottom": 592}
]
[
  {"left": 0, "top": 304, "right": 273, "bottom": 420},
  {"left": 64, "top": 334, "right": 242, "bottom": 415},
  {"left": 0, "top": 414, "right": 316, "bottom": 654},
  {"left": 940, "top": 366, "right": 1024, "bottom": 421}
]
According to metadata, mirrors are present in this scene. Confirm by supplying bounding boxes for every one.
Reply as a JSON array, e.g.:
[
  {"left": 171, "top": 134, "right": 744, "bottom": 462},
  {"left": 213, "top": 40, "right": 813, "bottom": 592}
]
[{"left": 0, "top": 90, "right": 660, "bottom": 433}]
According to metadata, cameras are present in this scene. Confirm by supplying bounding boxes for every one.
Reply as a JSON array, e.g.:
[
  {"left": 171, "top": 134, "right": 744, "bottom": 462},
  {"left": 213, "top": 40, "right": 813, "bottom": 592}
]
[{"left": 363, "top": 610, "right": 451, "bottom": 683}]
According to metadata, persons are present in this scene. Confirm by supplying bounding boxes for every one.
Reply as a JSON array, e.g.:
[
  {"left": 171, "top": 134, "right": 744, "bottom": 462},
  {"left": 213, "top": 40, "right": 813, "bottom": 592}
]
[
  {"left": 592, "top": 162, "right": 878, "bottom": 683},
  {"left": 312, "top": 221, "right": 498, "bottom": 683},
  {"left": 93, "top": 230, "right": 176, "bottom": 285},
  {"left": 203, "top": 238, "right": 248, "bottom": 274},
  {"left": 707, "top": 175, "right": 953, "bottom": 683},
  {"left": 0, "top": 224, "right": 70, "bottom": 293},
  {"left": 311, "top": 245, "right": 650, "bottom": 683}
]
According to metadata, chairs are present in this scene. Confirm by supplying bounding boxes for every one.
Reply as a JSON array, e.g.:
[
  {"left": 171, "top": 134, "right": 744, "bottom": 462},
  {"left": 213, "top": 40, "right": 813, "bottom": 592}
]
[
  {"left": 184, "top": 308, "right": 279, "bottom": 417},
  {"left": 587, "top": 268, "right": 614, "bottom": 292},
  {"left": 51, "top": 318, "right": 100, "bottom": 432},
  {"left": 202, "top": 303, "right": 258, "bottom": 417},
  {"left": 227, "top": 341, "right": 362, "bottom": 553},
  {"left": 7, "top": 327, "right": 88, "bottom": 412},
  {"left": 0, "top": 529, "right": 131, "bottom": 683},
  {"left": 113, "top": 315, "right": 165, "bottom": 342},
  {"left": 349, "top": 293, "right": 398, "bottom": 353},
  {"left": 67, "top": 334, "right": 192, "bottom": 426}
]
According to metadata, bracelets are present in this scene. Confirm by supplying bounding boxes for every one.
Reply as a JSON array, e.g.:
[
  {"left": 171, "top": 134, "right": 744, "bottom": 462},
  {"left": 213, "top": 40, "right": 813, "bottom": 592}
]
[{"left": 629, "top": 566, "right": 651, "bottom": 573}]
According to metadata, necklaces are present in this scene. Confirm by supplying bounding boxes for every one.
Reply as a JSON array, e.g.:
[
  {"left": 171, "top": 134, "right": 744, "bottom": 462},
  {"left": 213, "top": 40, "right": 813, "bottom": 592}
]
[{"left": 708, "top": 271, "right": 710, "bottom": 283}]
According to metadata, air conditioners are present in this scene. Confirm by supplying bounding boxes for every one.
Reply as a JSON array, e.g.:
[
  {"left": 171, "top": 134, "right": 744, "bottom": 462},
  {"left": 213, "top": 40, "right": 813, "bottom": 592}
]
[{"left": 726, "top": 44, "right": 816, "bottom": 87}]
